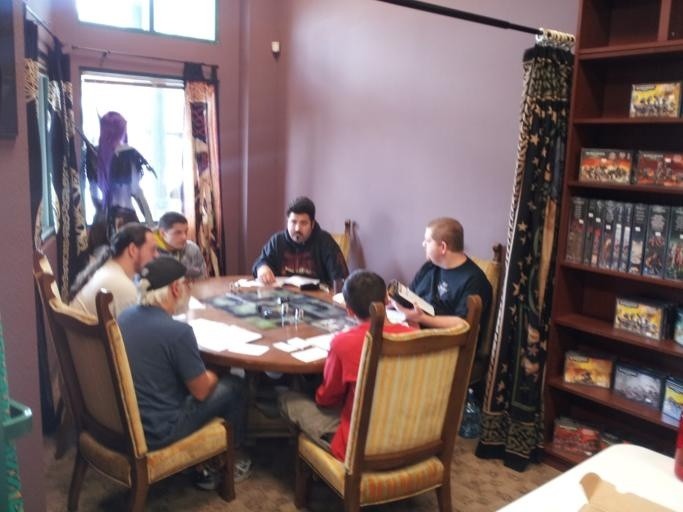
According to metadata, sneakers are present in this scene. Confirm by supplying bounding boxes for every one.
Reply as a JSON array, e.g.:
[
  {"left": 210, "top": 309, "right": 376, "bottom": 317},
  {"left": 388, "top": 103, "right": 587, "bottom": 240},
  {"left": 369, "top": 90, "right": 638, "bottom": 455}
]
[{"left": 195, "top": 457, "right": 253, "bottom": 491}]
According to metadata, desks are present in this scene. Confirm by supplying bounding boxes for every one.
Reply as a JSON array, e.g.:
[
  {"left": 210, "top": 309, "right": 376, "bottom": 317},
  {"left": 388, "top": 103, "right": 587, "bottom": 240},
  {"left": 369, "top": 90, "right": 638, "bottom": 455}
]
[
  {"left": 492, "top": 442, "right": 682, "bottom": 510},
  {"left": 174, "top": 274, "right": 423, "bottom": 450}
]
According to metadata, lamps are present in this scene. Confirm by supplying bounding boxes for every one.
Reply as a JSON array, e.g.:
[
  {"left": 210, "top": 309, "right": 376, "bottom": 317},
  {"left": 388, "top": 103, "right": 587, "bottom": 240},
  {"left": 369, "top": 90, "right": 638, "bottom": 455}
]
[{"left": 272, "top": 40, "right": 280, "bottom": 59}]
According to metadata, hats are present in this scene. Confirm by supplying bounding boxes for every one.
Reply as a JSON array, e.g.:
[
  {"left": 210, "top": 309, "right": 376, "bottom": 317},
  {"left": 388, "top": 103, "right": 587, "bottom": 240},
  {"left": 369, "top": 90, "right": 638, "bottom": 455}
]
[{"left": 137, "top": 257, "right": 202, "bottom": 292}]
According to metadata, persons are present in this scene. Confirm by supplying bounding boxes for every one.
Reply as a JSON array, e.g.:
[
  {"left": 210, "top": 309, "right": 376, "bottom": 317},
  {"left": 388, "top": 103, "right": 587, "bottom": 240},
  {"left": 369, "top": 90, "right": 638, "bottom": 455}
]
[
  {"left": 116, "top": 256, "right": 254, "bottom": 488},
  {"left": 388, "top": 218, "right": 493, "bottom": 349},
  {"left": 151, "top": 211, "right": 205, "bottom": 281},
  {"left": 85, "top": 111, "right": 157, "bottom": 247},
  {"left": 277, "top": 269, "right": 419, "bottom": 463},
  {"left": 68, "top": 223, "right": 159, "bottom": 321},
  {"left": 252, "top": 195, "right": 349, "bottom": 291}
]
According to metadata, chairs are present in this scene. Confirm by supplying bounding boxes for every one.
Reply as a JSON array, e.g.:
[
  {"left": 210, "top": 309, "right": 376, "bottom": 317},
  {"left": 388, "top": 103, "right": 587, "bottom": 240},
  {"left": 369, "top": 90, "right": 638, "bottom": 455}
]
[
  {"left": 32, "top": 249, "right": 80, "bottom": 460},
  {"left": 108, "top": 207, "right": 138, "bottom": 243},
  {"left": 469, "top": 254, "right": 501, "bottom": 393},
  {"left": 329, "top": 213, "right": 354, "bottom": 263},
  {"left": 39, "top": 272, "right": 235, "bottom": 511},
  {"left": 297, "top": 295, "right": 481, "bottom": 512}
]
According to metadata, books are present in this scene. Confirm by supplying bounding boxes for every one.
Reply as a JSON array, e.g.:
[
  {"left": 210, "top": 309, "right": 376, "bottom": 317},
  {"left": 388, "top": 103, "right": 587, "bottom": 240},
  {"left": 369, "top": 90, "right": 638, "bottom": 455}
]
[{"left": 564, "top": 195, "right": 683, "bottom": 278}]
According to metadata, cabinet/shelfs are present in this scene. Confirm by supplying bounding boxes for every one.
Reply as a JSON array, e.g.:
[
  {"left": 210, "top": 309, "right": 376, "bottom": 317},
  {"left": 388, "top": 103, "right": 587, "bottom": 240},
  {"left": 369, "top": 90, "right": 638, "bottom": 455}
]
[{"left": 538, "top": 0, "right": 682, "bottom": 464}]
